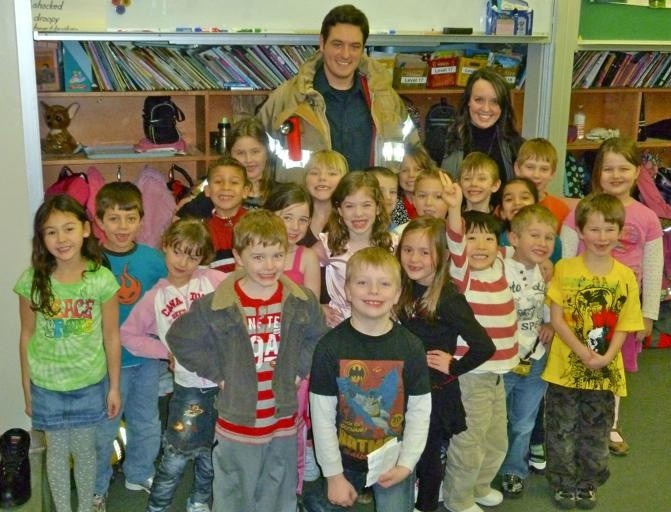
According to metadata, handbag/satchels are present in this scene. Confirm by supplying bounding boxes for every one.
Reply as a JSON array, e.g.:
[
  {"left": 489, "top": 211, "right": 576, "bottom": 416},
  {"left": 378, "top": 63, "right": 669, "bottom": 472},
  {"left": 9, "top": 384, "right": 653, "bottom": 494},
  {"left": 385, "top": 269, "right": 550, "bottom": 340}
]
[
  {"left": 43, "top": 166, "right": 90, "bottom": 207},
  {"left": 140, "top": 97, "right": 187, "bottom": 146}
]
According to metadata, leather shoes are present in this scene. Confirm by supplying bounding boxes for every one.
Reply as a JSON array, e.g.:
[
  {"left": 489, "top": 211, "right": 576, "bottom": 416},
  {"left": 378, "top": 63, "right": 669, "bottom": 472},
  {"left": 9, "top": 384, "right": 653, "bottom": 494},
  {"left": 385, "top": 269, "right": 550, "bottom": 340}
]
[
  {"left": 527, "top": 449, "right": 547, "bottom": 474},
  {"left": 608, "top": 426, "right": 630, "bottom": 457}
]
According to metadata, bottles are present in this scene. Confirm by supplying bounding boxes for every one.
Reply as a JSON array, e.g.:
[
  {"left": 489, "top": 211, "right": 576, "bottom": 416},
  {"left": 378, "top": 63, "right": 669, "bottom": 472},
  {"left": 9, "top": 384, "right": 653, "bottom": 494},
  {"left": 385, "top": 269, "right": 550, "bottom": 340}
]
[
  {"left": 573, "top": 104, "right": 587, "bottom": 143},
  {"left": 218, "top": 117, "right": 232, "bottom": 155}
]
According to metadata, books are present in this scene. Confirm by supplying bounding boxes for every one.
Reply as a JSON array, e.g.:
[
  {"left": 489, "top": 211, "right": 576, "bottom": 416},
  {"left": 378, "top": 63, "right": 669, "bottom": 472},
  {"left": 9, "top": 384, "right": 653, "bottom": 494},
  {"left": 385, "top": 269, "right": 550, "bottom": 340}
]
[
  {"left": 33, "top": 39, "right": 316, "bottom": 92},
  {"left": 570, "top": 49, "right": 670, "bottom": 90}
]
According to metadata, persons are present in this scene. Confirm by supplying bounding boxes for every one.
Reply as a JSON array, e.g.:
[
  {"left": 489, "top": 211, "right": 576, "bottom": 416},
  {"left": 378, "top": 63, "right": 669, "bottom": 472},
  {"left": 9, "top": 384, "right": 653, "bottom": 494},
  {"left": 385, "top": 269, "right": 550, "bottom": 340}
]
[
  {"left": 119, "top": 213, "right": 228, "bottom": 512},
  {"left": 460, "top": 152, "right": 500, "bottom": 215},
  {"left": 395, "top": 170, "right": 449, "bottom": 238},
  {"left": 514, "top": 135, "right": 572, "bottom": 237},
  {"left": 366, "top": 166, "right": 399, "bottom": 216},
  {"left": 438, "top": 169, "right": 555, "bottom": 512},
  {"left": 501, "top": 175, "right": 561, "bottom": 263},
  {"left": 13, "top": 192, "right": 121, "bottom": 512},
  {"left": 500, "top": 204, "right": 554, "bottom": 496},
  {"left": 441, "top": 66, "right": 527, "bottom": 201},
  {"left": 399, "top": 147, "right": 430, "bottom": 221},
  {"left": 203, "top": 156, "right": 250, "bottom": 276},
  {"left": 560, "top": 135, "right": 664, "bottom": 457},
  {"left": 263, "top": 181, "right": 321, "bottom": 512},
  {"left": 296, "top": 148, "right": 348, "bottom": 248},
  {"left": 177, "top": 116, "right": 275, "bottom": 218},
  {"left": 397, "top": 214, "right": 496, "bottom": 512},
  {"left": 545, "top": 194, "right": 646, "bottom": 510},
  {"left": 309, "top": 245, "right": 432, "bottom": 512},
  {"left": 318, "top": 170, "right": 399, "bottom": 330},
  {"left": 255, "top": 3, "right": 435, "bottom": 186},
  {"left": 93, "top": 182, "right": 169, "bottom": 494},
  {"left": 165, "top": 206, "right": 331, "bottom": 512}
]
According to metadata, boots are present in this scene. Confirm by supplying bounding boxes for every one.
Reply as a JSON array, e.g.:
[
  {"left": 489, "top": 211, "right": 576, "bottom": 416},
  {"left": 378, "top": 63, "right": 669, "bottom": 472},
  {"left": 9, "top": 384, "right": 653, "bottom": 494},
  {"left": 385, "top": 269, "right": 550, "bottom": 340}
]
[{"left": 0, "top": 428, "right": 33, "bottom": 508}]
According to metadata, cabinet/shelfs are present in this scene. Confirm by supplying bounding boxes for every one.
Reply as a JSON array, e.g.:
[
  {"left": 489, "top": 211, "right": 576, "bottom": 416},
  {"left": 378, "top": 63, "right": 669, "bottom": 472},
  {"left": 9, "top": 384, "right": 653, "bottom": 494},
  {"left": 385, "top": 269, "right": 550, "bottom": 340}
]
[
  {"left": 548, "top": 0, "right": 671, "bottom": 198},
  {"left": 0, "top": 0, "right": 550, "bottom": 244}
]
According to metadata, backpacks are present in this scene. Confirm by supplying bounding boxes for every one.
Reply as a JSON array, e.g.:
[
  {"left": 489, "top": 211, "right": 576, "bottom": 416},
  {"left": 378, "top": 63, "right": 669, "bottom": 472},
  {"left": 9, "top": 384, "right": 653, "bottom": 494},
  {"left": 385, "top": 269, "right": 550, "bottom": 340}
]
[{"left": 424, "top": 97, "right": 457, "bottom": 165}]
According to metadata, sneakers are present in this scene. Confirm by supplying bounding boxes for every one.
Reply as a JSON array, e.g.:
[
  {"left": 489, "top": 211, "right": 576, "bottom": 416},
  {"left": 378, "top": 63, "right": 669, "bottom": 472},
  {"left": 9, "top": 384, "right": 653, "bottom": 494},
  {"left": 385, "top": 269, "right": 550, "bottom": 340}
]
[
  {"left": 302, "top": 447, "right": 321, "bottom": 483},
  {"left": 476, "top": 486, "right": 503, "bottom": 507},
  {"left": 444, "top": 501, "right": 485, "bottom": 512},
  {"left": 124, "top": 472, "right": 155, "bottom": 495},
  {"left": 185, "top": 496, "right": 211, "bottom": 512},
  {"left": 501, "top": 475, "right": 525, "bottom": 499},
  {"left": 553, "top": 489, "right": 577, "bottom": 509},
  {"left": 577, "top": 490, "right": 597, "bottom": 511},
  {"left": 91, "top": 493, "right": 108, "bottom": 512}
]
